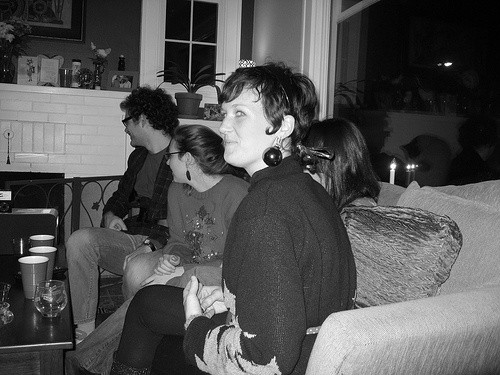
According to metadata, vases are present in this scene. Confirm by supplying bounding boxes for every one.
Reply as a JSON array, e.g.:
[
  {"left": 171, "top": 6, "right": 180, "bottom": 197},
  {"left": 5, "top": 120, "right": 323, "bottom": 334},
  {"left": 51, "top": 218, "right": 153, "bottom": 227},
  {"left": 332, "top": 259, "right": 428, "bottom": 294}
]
[
  {"left": 93, "top": 61, "right": 104, "bottom": 90},
  {"left": 0, "top": 39, "right": 15, "bottom": 83}
]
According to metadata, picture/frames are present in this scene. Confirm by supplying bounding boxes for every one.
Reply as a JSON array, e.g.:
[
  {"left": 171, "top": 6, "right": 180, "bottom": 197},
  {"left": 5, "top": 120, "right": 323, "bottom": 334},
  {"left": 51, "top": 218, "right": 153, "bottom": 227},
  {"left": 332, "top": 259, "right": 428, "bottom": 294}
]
[
  {"left": 20, "top": 0, "right": 86, "bottom": 44},
  {"left": 107, "top": 70, "right": 139, "bottom": 92}
]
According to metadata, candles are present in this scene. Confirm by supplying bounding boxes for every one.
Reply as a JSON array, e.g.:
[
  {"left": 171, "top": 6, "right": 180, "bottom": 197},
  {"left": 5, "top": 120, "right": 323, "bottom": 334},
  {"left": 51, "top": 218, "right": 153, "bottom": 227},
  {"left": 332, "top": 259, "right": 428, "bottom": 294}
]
[
  {"left": 389, "top": 157, "right": 396, "bottom": 184},
  {"left": 405, "top": 164, "right": 416, "bottom": 188}
]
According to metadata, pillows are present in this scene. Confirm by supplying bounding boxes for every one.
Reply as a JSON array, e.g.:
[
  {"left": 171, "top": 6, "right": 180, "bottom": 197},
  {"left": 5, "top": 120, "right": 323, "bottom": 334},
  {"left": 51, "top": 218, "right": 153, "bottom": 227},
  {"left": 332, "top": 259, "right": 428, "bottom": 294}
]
[
  {"left": 342, "top": 206, "right": 463, "bottom": 310},
  {"left": 395, "top": 181, "right": 500, "bottom": 294}
]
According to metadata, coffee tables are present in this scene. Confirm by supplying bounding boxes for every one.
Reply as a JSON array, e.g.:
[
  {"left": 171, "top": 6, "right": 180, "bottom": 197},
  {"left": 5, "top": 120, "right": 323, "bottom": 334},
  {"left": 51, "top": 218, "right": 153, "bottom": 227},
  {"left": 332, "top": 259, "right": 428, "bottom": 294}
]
[{"left": 0, "top": 246, "right": 74, "bottom": 375}]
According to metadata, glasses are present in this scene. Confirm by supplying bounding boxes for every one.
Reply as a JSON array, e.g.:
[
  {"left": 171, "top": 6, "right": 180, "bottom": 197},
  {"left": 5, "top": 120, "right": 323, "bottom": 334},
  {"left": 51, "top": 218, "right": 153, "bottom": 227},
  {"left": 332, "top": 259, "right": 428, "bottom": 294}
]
[
  {"left": 122, "top": 112, "right": 140, "bottom": 128},
  {"left": 166, "top": 151, "right": 187, "bottom": 160}
]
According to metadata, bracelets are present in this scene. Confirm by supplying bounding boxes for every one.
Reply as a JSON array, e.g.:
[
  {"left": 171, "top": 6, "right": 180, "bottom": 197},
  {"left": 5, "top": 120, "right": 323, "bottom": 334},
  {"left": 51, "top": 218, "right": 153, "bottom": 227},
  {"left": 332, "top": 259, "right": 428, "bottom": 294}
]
[{"left": 183, "top": 314, "right": 204, "bottom": 328}]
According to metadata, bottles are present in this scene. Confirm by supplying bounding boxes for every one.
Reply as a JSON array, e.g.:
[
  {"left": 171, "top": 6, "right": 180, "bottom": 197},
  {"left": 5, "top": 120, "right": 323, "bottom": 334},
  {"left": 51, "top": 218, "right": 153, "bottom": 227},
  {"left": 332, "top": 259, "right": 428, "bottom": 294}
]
[
  {"left": 70, "top": 59, "right": 82, "bottom": 87},
  {"left": 118, "top": 55, "right": 126, "bottom": 70}
]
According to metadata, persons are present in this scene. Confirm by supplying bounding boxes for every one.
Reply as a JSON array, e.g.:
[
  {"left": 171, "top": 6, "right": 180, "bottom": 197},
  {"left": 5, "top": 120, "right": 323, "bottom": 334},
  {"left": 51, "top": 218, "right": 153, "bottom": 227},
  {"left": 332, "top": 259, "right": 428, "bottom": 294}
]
[
  {"left": 64, "top": 117, "right": 378, "bottom": 375},
  {"left": 111, "top": 66, "right": 356, "bottom": 375},
  {"left": 124, "top": 125, "right": 250, "bottom": 300},
  {"left": 64, "top": 87, "right": 179, "bottom": 346}
]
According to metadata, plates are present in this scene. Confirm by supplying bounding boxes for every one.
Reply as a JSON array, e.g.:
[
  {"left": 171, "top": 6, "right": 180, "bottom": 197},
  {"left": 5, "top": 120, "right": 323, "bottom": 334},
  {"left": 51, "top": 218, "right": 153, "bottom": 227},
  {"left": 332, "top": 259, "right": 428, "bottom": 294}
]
[{"left": 53, "top": 261, "right": 67, "bottom": 275}]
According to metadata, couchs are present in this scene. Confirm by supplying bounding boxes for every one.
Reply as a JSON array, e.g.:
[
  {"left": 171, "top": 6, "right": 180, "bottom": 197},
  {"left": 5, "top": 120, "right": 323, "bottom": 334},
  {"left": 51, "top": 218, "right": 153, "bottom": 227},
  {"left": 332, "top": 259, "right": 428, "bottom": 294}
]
[{"left": 302, "top": 182, "right": 500, "bottom": 375}]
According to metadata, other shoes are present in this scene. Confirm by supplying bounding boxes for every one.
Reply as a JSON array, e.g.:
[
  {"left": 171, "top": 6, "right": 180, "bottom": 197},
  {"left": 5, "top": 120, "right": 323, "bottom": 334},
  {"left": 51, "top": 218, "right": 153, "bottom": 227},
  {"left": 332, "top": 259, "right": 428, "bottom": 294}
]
[{"left": 75, "top": 328, "right": 89, "bottom": 345}]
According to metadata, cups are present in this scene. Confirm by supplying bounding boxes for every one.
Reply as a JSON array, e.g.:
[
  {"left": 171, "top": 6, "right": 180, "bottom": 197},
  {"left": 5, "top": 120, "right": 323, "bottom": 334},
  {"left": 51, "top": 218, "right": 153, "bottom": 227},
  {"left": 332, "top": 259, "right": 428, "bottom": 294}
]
[
  {"left": 58, "top": 68, "right": 72, "bottom": 87},
  {"left": 13, "top": 237, "right": 29, "bottom": 274},
  {"left": 0, "top": 282, "right": 14, "bottom": 328},
  {"left": 29, "top": 235, "right": 55, "bottom": 248},
  {"left": 18, "top": 256, "right": 49, "bottom": 300},
  {"left": 33, "top": 280, "right": 68, "bottom": 318},
  {"left": 29, "top": 246, "right": 57, "bottom": 280}
]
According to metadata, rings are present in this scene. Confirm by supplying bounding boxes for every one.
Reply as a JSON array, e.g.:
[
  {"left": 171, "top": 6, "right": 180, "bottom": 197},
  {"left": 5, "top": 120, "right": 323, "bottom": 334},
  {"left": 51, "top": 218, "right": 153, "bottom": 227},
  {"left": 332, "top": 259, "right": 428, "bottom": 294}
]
[{"left": 115, "top": 225, "right": 118, "bottom": 229}]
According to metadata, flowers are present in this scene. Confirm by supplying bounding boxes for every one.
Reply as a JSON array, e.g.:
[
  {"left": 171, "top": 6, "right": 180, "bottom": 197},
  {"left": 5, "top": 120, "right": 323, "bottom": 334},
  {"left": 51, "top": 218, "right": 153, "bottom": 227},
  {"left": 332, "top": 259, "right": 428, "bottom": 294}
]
[
  {"left": 88, "top": 41, "right": 111, "bottom": 63},
  {"left": 0, "top": 15, "right": 33, "bottom": 58}
]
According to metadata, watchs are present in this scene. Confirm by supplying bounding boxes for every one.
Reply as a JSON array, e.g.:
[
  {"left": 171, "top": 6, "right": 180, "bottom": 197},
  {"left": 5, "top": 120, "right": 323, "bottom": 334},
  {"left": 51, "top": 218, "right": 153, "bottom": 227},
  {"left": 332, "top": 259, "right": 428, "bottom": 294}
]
[{"left": 143, "top": 240, "right": 156, "bottom": 251}]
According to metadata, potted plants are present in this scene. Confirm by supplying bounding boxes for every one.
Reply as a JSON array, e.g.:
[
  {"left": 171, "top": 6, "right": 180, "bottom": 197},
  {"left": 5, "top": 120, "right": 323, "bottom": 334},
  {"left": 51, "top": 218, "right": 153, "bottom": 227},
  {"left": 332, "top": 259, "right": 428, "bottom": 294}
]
[{"left": 155, "top": 64, "right": 226, "bottom": 117}]
